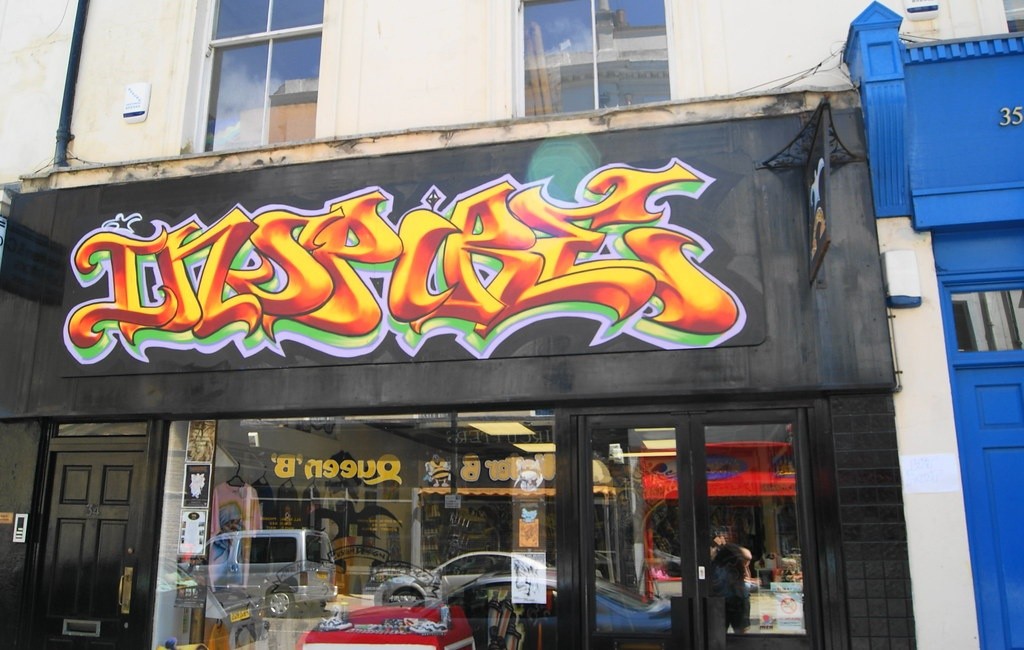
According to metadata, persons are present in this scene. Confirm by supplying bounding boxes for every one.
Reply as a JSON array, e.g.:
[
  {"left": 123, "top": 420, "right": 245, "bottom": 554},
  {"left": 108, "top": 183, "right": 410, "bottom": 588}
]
[{"left": 711, "top": 527, "right": 752, "bottom": 633}]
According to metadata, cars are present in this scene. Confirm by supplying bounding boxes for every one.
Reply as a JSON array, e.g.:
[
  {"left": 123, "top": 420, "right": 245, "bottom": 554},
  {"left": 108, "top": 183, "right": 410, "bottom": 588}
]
[
  {"left": 412, "top": 568, "right": 672, "bottom": 650},
  {"left": 159, "top": 557, "right": 271, "bottom": 650},
  {"left": 373, "top": 551, "right": 548, "bottom": 608}
]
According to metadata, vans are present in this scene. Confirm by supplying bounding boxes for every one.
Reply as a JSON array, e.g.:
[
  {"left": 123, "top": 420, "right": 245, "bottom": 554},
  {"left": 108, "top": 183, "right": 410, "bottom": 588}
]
[
  {"left": 294, "top": 603, "right": 475, "bottom": 650},
  {"left": 175, "top": 528, "right": 338, "bottom": 620}
]
